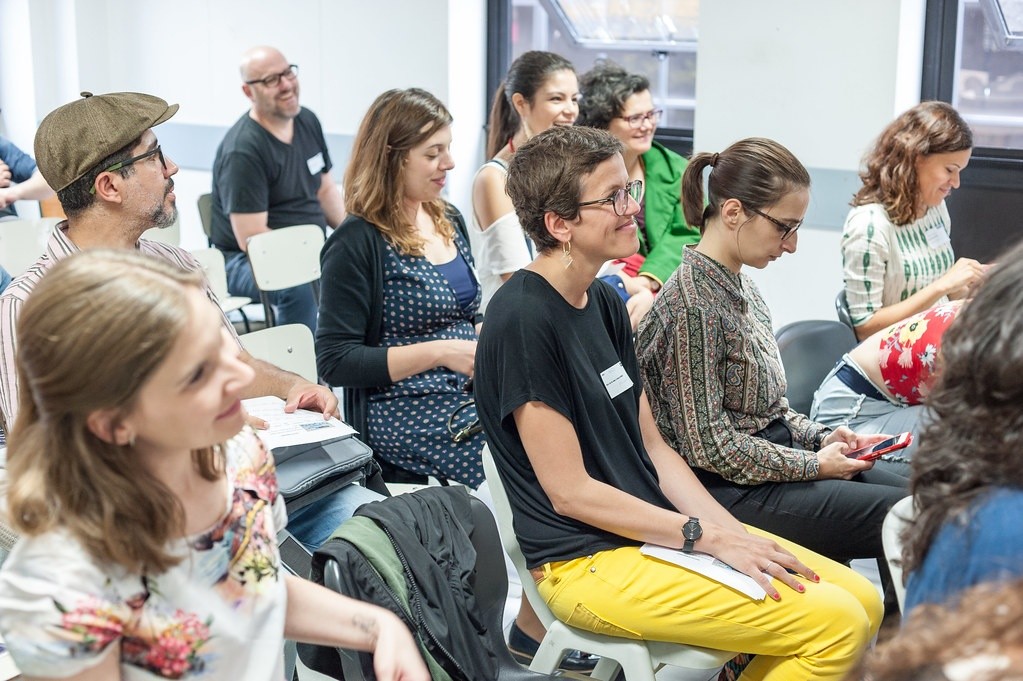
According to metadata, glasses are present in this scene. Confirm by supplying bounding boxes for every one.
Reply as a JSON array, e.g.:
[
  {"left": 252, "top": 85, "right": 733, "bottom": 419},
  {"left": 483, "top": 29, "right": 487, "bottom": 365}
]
[
  {"left": 89, "top": 144, "right": 167, "bottom": 197},
  {"left": 246, "top": 63, "right": 299, "bottom": 89},
  {"left": 615, "top": 108, "right": 663, "bottom": 129},
  {"left": 720, "top": 199, "right": 804, "bottom": 242},
  {"left": 576, "top": 180, "right": 643, "bottom": 218}
]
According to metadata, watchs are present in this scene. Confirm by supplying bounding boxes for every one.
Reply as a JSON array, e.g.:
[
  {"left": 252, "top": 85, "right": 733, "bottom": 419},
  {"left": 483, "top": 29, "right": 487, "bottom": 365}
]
[{"left": 681, "top": 516, "right": 704, "bottom": 553}]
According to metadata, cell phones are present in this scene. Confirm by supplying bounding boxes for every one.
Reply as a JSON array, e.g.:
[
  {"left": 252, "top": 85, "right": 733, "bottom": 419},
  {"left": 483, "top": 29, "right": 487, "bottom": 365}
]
[
  {"left": 275, "top": 529, "right": 314, "bottom": 580},
  {"left": 844, "top": 431, "right": 911, "bottom": 460}
]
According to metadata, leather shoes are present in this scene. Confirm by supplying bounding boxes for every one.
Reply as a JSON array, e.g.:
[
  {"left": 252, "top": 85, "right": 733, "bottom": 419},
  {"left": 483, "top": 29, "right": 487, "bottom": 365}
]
[{"left": 507, "top": 618, "right": 599, "bottom": 673}]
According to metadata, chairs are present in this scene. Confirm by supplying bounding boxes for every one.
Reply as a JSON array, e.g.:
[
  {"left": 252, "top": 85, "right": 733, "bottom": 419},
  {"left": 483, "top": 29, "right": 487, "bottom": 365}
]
[
  {"left": 322, "top": 492, "right": 605, "bottom": 681},
  {"left": 481, "top": 443, "right": 741, "bottom": 681},
  {"left": 239, "top": 323, "right": 317, "bottom": 384},
  {"left": 246, "top": 225, "right": 344, "bottom": 424},
  {"left": 774, "top": 292, "right": 900, "bottom": 614},
  {"left": 197, "top": 192, "right": 277, "bottom": 330}
]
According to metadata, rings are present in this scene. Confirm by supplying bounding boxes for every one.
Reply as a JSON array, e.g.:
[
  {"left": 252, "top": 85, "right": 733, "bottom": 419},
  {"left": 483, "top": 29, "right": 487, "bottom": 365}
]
[{"left": 765, "top": 562, "right": 772, "bottom": 571}]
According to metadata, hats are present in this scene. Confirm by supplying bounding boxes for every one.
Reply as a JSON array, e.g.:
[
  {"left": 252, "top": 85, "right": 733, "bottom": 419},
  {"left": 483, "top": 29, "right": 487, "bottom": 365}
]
[{"left": 34, "top": 91, "right": 179, "bottom": 193}]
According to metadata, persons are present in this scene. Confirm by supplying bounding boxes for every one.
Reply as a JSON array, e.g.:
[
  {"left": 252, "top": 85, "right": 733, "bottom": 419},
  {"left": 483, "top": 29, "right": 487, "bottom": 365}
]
[
  {"left": 473, "top": 51, "right": 704, "bottom": 342},
  {"left": 0, "top": 135, "right": 55, "bottom": 223},
  {"left": 0, "top": 246, "right": 430, "bottom": 681},
  {"left": 636, "top": 139, "right": 923, "bottom": 647},
  {"left": 808, "top": 297, "right": 983, "bottom": 487},
  {"left": 471, "top": 127, "right": 885, "bottom": 681},
  {"left": 206, "top": 48, "right": 349, "bottom": 392},
  {"left": 899, "top": 238, "right": 1023, "bottom": 681},
  {"left": 1, "top": 93, "right": 395, "bottom": 555},
  {"left": 841, "top": 102, "right": 1002, "bottom": 340},
  {"left": 316, "top": 86, "right": 624, "bottom": 676}
]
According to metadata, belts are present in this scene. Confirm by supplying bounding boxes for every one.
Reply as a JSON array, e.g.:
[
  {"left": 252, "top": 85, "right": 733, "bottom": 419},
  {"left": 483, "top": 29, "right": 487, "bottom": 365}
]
[{"left": 833, "top": 358, "right": 888, "bottom": 402}]
